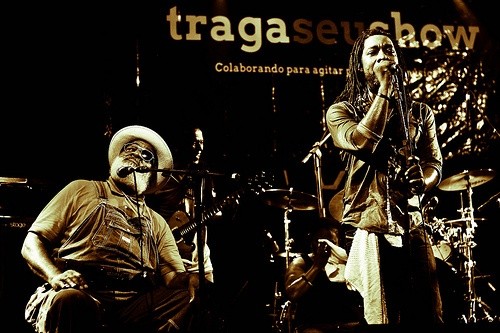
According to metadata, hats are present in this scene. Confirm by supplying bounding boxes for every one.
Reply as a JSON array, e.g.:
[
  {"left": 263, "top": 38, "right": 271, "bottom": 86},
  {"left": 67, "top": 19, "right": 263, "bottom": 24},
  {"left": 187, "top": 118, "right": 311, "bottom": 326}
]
[{"left": 108, "top": 125, "right": 174, "bottom": 195}]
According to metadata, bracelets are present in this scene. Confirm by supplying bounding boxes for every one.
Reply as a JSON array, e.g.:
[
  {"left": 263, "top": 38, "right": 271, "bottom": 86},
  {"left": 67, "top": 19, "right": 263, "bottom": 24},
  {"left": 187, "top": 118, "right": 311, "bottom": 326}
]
[{"left": 376, "top": 92, "right": 391, "bottom": 102}]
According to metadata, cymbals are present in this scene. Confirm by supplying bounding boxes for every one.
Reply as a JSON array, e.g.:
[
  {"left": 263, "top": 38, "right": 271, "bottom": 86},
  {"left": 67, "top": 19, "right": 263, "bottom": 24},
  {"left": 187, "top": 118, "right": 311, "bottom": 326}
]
[
  {"left": 262, "top": 189, "right": 318, "bottom": 210},
  {"left": 440, "top": 166, "right": 492, "bottom": 191}
]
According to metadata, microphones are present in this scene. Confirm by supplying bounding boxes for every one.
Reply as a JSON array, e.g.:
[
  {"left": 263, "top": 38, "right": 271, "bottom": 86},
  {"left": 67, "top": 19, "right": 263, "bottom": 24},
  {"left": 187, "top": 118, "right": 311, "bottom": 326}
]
[
  {"left": 264, "top": 229, "right": 279, "bottom": 252},
  {"left": 390, "top": 63, "right": 400, "bottom": 71},
  {"left": 117, "top": 166, "right": 133, "bottom": 178}
]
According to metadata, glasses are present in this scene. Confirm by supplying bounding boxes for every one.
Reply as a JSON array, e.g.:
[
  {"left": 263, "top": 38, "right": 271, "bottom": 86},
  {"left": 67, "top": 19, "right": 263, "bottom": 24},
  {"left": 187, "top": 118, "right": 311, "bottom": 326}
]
[{"left": 123, "top": 142, "right": 155, "bottom": 163}]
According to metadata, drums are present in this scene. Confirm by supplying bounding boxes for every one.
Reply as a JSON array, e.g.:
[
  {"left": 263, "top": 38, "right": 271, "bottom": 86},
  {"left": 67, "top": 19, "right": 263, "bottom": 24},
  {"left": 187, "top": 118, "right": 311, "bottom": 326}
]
[{"left": 433, "top": 242, "right": 453, "bottom": 260}]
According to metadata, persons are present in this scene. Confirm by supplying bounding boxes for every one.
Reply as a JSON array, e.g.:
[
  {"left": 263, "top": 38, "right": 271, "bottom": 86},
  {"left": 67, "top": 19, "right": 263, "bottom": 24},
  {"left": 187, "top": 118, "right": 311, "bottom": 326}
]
[
  {"left": 21, "top": 125, "right": 201, "bottom": 333},
  {"left": 326, "top": 29, "right": 444, "bottom": 324},
  {"left": 168, "top": 128, "right": 214, "bottom": 283}
]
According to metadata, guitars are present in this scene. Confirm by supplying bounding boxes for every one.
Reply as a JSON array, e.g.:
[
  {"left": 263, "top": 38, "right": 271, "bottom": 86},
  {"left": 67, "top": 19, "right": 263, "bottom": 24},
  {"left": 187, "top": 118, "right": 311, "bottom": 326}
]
[{"left": 170, "top": 174, "right": 267, "bottom": 254}]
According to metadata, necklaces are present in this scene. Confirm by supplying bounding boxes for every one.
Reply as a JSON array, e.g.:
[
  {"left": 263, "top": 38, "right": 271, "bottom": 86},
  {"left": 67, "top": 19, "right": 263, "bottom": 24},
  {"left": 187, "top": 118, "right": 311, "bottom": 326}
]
[{"left": 122, "top": 192, "right": 153, "bottom": 236}]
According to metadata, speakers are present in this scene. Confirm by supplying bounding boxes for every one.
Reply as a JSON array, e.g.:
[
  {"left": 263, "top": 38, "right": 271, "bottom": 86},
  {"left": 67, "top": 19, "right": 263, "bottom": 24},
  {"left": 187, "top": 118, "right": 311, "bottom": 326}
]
[{"left": 0, "top": 216, "right": 45, "bottom": 333}]
